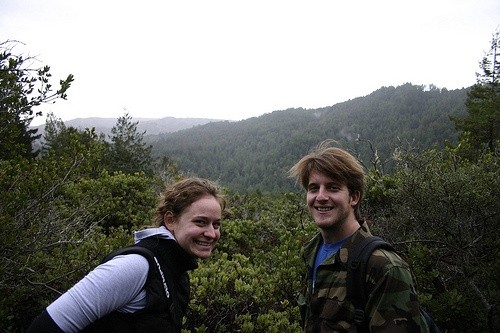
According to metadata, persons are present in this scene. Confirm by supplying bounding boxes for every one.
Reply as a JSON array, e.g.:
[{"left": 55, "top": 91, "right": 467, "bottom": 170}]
[
  {"left": 27, "top": 177, "right": 226, "bottom": 333},
  {"left": 289, "top": 139, "right": 429, "bottom": 333}
]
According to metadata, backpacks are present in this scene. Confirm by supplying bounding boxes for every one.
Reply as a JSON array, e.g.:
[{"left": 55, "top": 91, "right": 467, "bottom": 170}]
[{"left": 346, "top": 236, "right": 439, "bottom": 333}]
